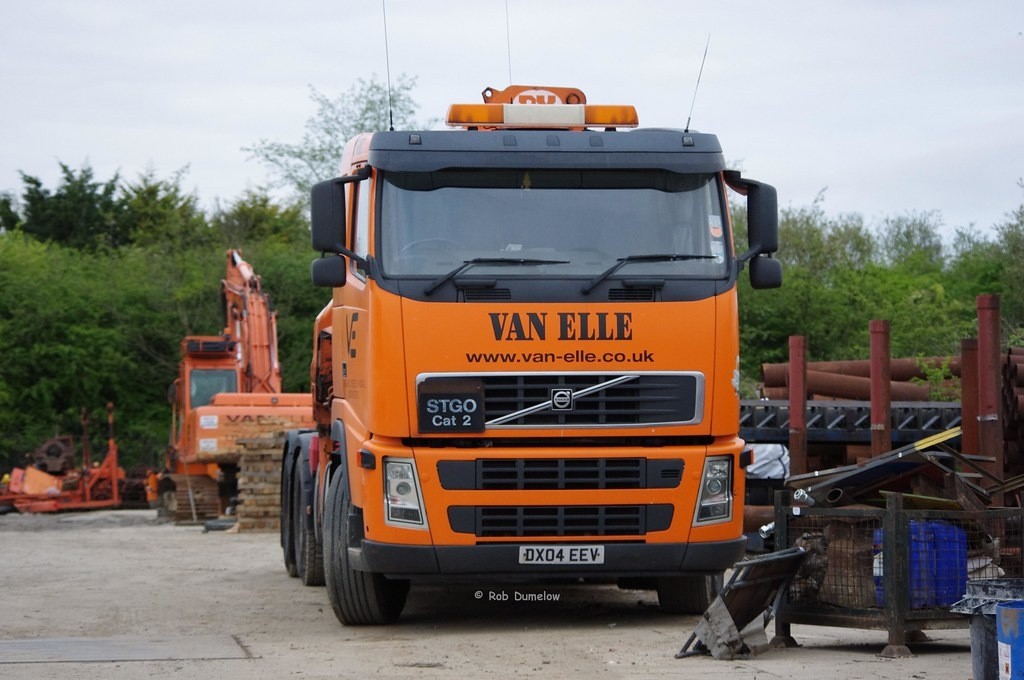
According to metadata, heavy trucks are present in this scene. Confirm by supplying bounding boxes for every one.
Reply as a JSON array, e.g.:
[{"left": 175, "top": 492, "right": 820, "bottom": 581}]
[{"left": 280, "top": 85, "right": 783, "bottom": 627}]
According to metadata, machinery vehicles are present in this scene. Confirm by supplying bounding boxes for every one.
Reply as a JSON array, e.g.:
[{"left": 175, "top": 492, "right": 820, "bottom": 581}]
[{"left": 157, "top": 248, "right": 317, "bottom": 534}]
